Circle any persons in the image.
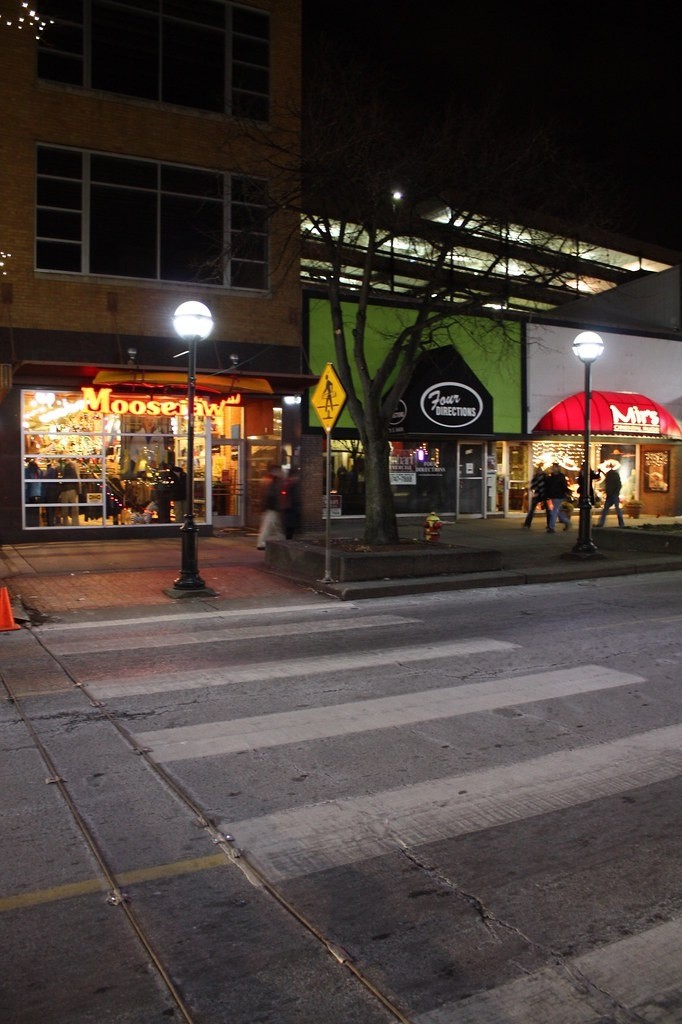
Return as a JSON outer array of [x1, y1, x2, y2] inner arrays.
[[592, 463, 625, 527], [578, 462, 600, 505], [546, 463, 571, 534], [256, 465, 303, 551], [25, 459, 186, 526], [524, 465, 551, 529]]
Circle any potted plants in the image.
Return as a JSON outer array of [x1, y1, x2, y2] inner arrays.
[[624, 500, 643, 520], [558, 502, 574, 523]]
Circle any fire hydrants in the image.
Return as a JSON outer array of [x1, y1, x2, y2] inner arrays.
[[421, 512, 443, 543]]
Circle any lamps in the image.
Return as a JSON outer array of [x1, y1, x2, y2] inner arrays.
[[230, 353, 239, 370], [127, 348, 138, 364]]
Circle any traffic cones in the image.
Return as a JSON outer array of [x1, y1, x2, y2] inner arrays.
[[1, 585, 22, 631]]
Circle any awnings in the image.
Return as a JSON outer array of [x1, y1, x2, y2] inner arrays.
[[533, 391, 682, 439], [11, 363, 321, 396]]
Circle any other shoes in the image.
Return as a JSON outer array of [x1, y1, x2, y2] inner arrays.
[[562, 523, 572, 532], [547, 528, 556, 533], [522, 524, 532, 529], [257, 544, 266, 550]]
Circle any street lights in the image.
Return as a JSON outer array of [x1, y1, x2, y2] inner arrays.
[[567, 330, 606, 554], [171, 300, 216, 598]]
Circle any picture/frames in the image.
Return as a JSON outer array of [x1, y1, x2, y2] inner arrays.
[[231, 424, 240, 460]]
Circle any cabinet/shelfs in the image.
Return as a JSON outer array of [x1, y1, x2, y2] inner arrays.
[[194, 478, 206, 504]]
